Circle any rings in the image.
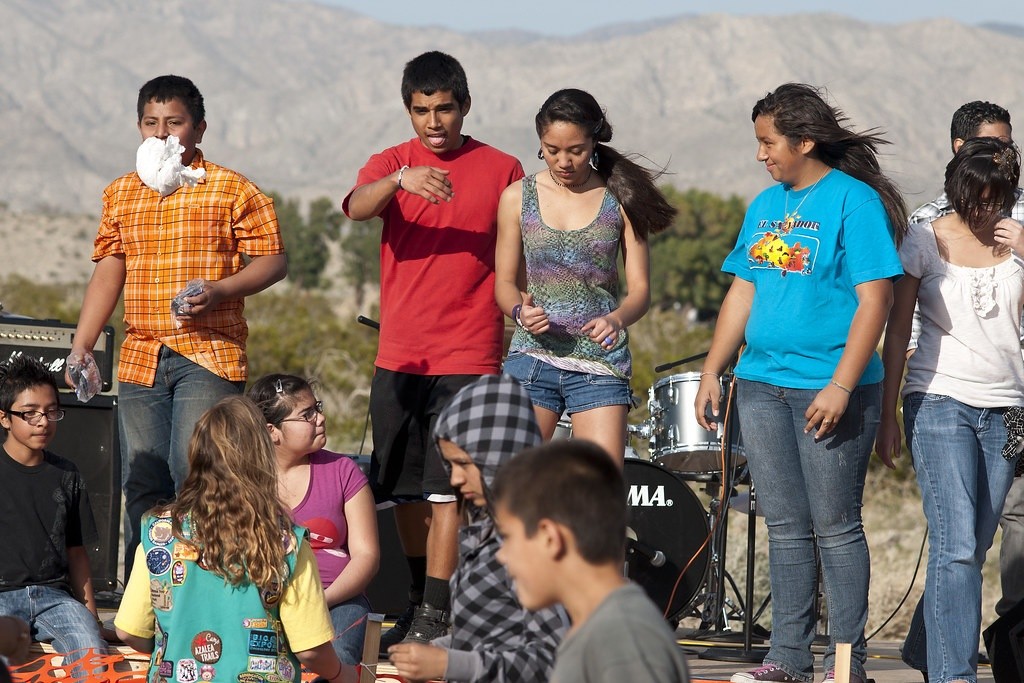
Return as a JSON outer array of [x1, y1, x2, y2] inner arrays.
[[823, 418, 831, 428], [604, 337, 613, 346]]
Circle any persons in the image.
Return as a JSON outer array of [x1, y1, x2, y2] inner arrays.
[[388, 374, 572, 683], [491, 437, 691, 683], [494, 86, 679, 470], [342, 50, 525, 660], [63, 75, 287, 584], [905, 101, 1024, 683], [0, 358, 107, 683], [113, 396, 359, 683], [249, 374, 379, 683], [694, 85, 907, 683], [875, 136, 1024, 683]]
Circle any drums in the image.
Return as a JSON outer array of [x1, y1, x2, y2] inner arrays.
[[621, 456, 712, 624], [648, 371, 747, 475], [549, 418, 632, 446]]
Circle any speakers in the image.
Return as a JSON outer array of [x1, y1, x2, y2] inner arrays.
[[47, 386, 122, 593]]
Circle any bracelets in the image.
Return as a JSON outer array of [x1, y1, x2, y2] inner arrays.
[[399, 165, 409, 190], [329, 662, 341, 680], [700, 372, 720, 379], [831, 381, 851, 395], [512, 304, 529, 332]]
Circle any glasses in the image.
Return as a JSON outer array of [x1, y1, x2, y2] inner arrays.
[[3, 410, 66, 423], [281, 401, 323, 422], [960, 197, 1004, 213]]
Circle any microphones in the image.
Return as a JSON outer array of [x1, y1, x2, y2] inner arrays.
[[627, 538, 666, 568]]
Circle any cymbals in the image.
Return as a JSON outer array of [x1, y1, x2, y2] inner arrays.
[[730, 492, 765, 517], [502, 325, 515, 358]]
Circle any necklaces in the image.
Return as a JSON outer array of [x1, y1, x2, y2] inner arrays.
[[550, 164, 592, 188], [782, 165, 831, 231]]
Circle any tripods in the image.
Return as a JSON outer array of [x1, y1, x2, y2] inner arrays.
[[668, 475, 772, 642]]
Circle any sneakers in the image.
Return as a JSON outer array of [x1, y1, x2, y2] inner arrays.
[[730, 665, 804, 683], [821, 669, 864, 683], [378, 605, 418, 658], [400, 603, 452, 645]]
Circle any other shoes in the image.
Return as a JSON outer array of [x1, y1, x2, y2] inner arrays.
[[975, 651, 991, 666]]
